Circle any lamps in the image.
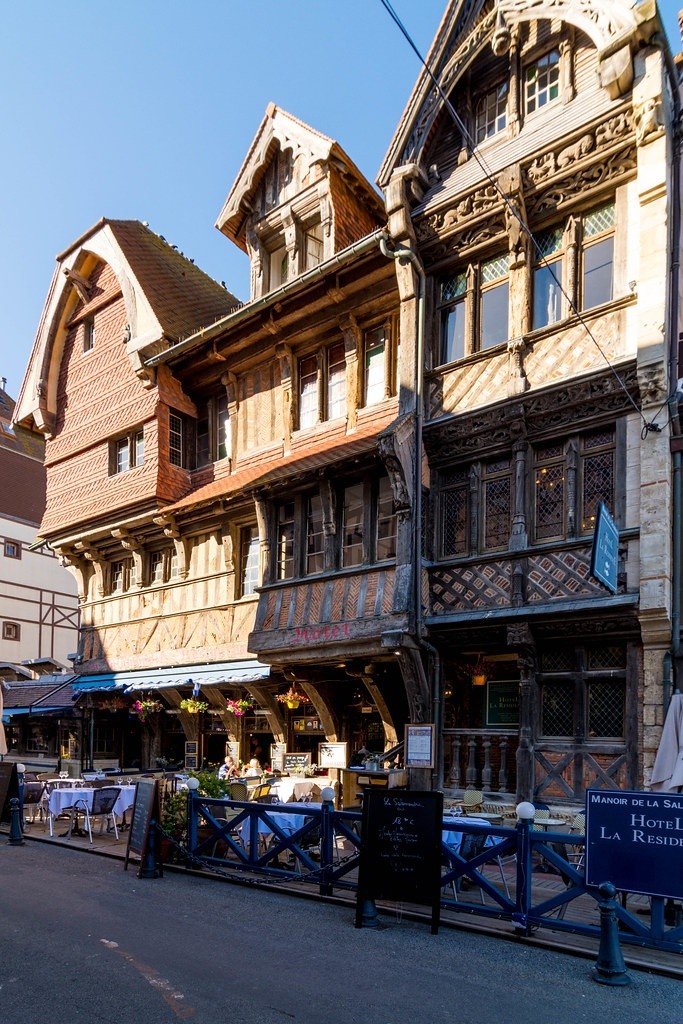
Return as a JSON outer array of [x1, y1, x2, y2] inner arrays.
[[187, 778, 200, 789], [516, 802, 536, 819], [17, 764, 26, 773], [321, 787, 335, 801]]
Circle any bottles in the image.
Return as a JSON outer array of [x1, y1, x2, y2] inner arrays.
[[96, 768, 102, 776]]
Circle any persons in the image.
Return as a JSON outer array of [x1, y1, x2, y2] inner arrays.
[[219, 756, 240, 782], [241, 759, 263, 778]]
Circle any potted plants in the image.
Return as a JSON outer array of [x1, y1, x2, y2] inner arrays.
[[98, 698, 127, 712], [159, 767, 232, 861], [180, 698, 208, 714]]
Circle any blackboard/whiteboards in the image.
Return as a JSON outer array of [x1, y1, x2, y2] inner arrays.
[[281, 752, 311, 773], [317, 742, 347, 769], [271, 760, 282, 772], [128, 777, 162, 858], [0, 761, 25, 831], [226, 742, 239, 769], [403, 722, 436, 768], [270, 743, 286, 759], [185, 756, 198, 768], [359, 785, 443, 906], [184, 741, 199, 754], [585, 790, 683, 902]]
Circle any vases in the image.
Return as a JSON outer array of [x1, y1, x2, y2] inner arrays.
[[287, 701, 300, 709], [147, 706, 155, 713]]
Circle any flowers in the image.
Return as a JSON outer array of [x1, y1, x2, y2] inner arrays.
[[275, 686, 310, 704], [240, 760, 250, 772], [133, 697, 164, 723], [225, 698, 255, 716]]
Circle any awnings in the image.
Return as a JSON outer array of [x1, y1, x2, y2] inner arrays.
[[2, 707, 66, 723]]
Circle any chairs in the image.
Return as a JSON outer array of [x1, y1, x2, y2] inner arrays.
[[229, 776, 585, 915], [23, 766, 186, 844]]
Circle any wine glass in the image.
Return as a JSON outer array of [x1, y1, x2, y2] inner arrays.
[[117, 777, 123, 787], [450, 805, 462, 822], [60, 770, 69, 779], [73, 780, 85, 789], [127, 777, 132, 787], [270, 797, 283, 806], [301, 792, 313, 805]]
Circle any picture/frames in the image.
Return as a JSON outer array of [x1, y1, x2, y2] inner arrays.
[[317, 743, 348, 770]]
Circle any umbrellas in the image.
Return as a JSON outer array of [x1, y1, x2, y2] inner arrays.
[[649, 689, 683, 792]]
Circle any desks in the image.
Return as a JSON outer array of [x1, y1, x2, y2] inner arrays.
[[47, 788, 105, 837], [83, 774, 104, 781], [240, 800, 339, 872], [46, 778, 85, 789], [103, 784, 137, 831], [247, 783, 263, 790], [443, 816, 491, 892], [535, 818, 565, 872]]
